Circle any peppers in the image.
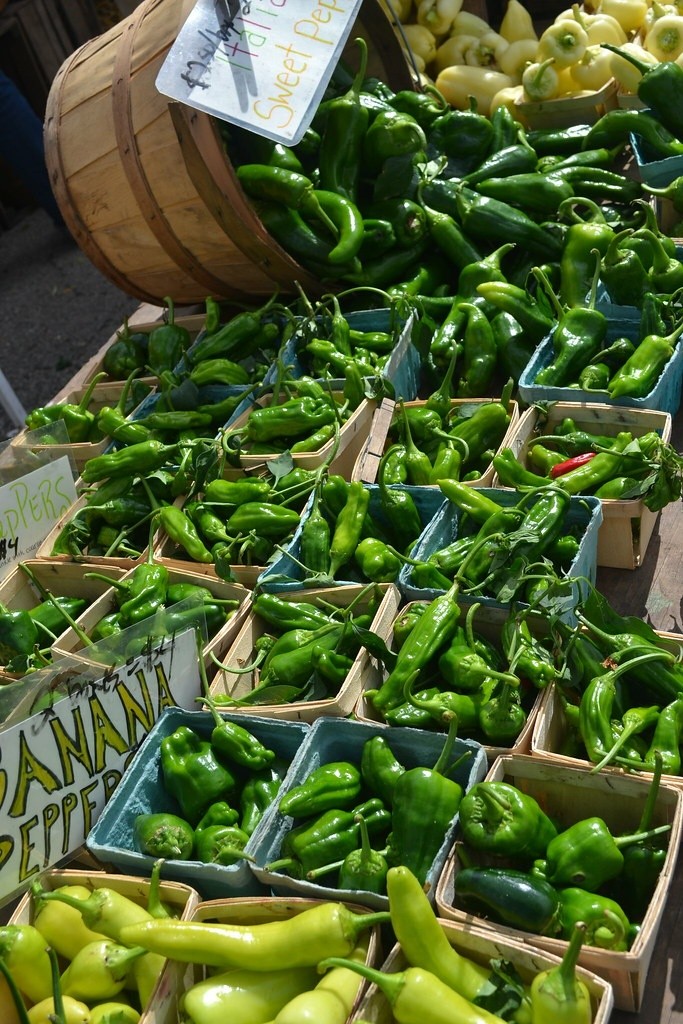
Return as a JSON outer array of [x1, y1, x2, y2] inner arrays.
[[0, 0, 683, 1024]]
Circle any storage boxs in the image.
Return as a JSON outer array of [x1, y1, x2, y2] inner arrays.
[[0, 41, 683, 1024]]
